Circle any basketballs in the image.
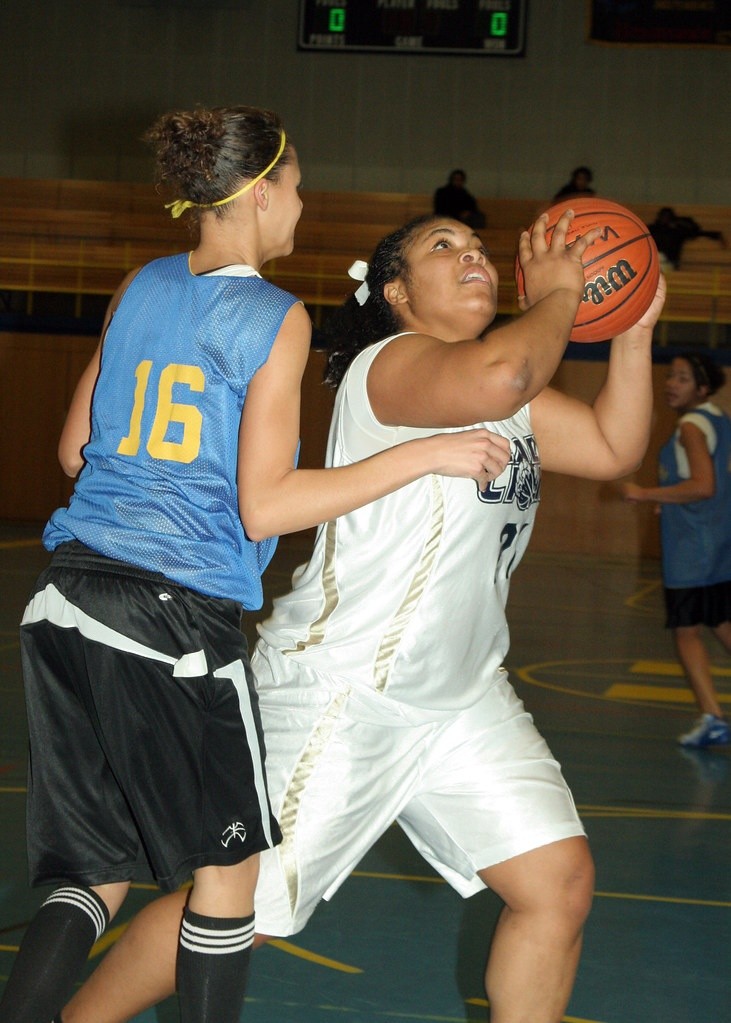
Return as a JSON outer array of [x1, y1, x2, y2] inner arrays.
[[512, 195, 660, 344]]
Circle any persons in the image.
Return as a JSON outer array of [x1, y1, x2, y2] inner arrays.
[[45, 216, 666, 1023], [432, 169, 485, 229], [618, 350, 731, 747], [0, 105, 510, 1023], [647, 207, 683, 270], [550, 166, 595, 204]]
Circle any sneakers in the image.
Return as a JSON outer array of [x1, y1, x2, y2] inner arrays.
[[677, 713, 731, 746]]
